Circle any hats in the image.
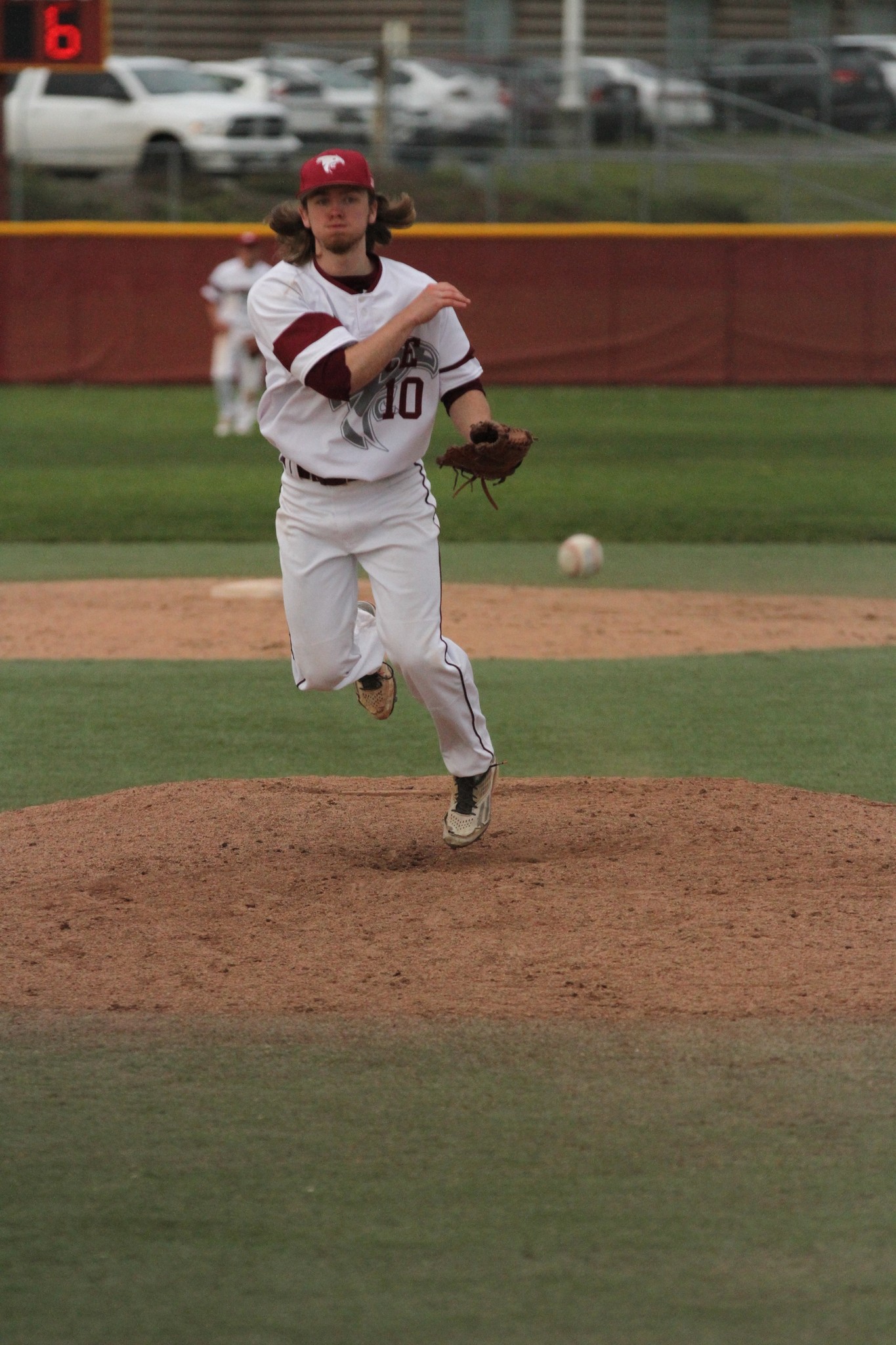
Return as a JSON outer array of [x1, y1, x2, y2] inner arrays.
[[240, 231, 259, 245], [300, 149, 376, 194]]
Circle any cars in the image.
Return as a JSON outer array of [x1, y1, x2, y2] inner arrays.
[[684, 36, 896, 137], [0, 54, 305, 180], [195, 54, 511, 148], [511, 56, 714, 147]]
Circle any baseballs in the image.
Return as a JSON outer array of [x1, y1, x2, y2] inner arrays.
[[558, 533, 605, 582]]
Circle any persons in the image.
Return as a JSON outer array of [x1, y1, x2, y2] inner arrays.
[[247, 150, 532, 846], [202, 229, 273, 438]]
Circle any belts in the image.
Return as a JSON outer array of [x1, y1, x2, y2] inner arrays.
[[282, 457, 358, 485]]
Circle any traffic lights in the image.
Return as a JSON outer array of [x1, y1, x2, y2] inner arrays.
[[0, 0, 114, 75]]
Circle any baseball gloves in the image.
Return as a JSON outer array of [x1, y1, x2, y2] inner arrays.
[[436, 419, 535, 482], [246, 335, 261, 360]]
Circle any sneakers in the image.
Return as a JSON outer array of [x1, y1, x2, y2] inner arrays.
[[442, 758, 507, 848], [353, 601, 397, 720]]
